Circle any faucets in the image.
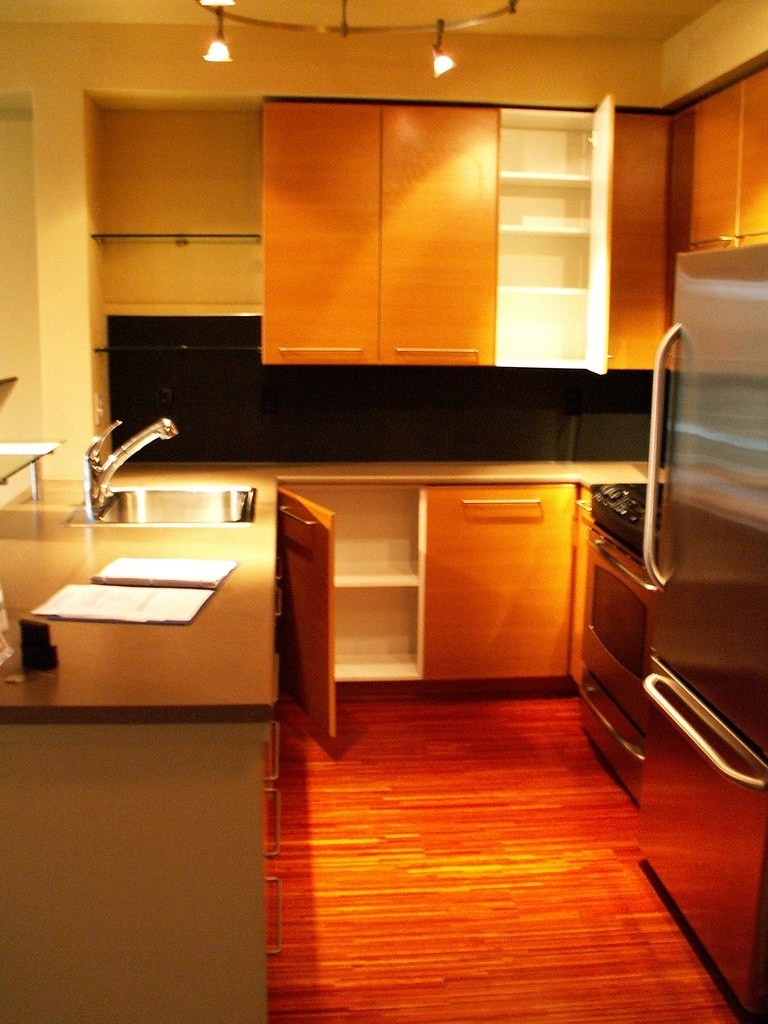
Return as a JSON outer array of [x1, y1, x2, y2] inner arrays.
[[82, 417, 180, 510]]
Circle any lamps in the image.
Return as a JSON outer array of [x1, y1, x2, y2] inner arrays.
[[429, 20, 456, 79], [203, 6, 234, 62]]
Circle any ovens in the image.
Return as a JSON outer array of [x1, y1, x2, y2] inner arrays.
[[578, 481, 663, 804]]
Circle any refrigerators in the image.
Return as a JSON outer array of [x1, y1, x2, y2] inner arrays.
[[636, 244, 767, 1024]]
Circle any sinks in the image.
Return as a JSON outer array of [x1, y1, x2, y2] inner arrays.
[[59, 483, 258, 530]]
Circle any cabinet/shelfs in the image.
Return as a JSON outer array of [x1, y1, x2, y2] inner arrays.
[[668, 67, 768, 245], [495, 92, 669, 375], [260, 103, 502, 366], [278, 483, 582, 738]]
